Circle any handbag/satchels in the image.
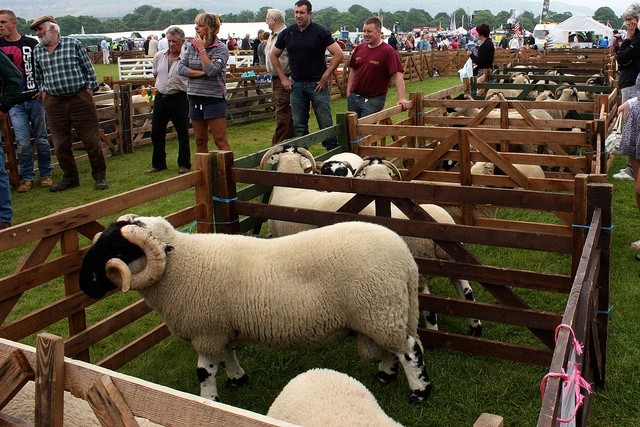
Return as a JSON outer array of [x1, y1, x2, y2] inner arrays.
[[605, 129, 622, 156]]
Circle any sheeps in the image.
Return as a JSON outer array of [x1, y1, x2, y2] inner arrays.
[[94, 80, 161, 120], [489, 50, 609, 147], [319, 150, 365, 180], [446, 92, 565, 171], [442, 141, 497, 174], [77, 213, 433, 405], [468, 155, 548, 198], [352, 155, 482, 336], [258, 143, 374, 238]]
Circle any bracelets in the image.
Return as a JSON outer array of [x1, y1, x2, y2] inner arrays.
[[198, 49, 206, 55]]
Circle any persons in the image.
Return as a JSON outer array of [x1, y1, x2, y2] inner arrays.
[[237, 36, 242, 49], [269, 0, 344, 152], [177, 13, 230, 153], [347, 17, 411, 138], [143, 36, 153, 55], [388, 33, 482, 51], [252, 29, 265, 66], [529, 34, 535, 48], [1, 10, 53, 193], [509, 35, 524, 49], [257, 32, 270, 65], [600, 36, 609, 48], [30, 17, 109, 192], [467, 24, 496, 77], [543, 29, 554, 49], [612, 3, 640, 181], [242, 33, 252, 50], [616, 74, 640, 258], [610, 31, 626, 73], [266, 9, 294, 146], [0, 49, 22, 230], [148, 35, 159, 55], [112, 37, 134, 51], [498, 34, 508, 48], [158, 32, 168, 51], [101, 36, 111, 65], [593, 34, 601, 47], [227, 35, 237, 50], [145, 26, 191, 174]]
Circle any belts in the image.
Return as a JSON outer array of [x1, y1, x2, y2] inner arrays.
[[272, 74, 290, 79]]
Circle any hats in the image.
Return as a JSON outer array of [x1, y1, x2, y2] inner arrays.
[[30, 16, 56, 30]]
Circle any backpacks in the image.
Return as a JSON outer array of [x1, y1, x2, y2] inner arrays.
[[615, 35, 623, 48]]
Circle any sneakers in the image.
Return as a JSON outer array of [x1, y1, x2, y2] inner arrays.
[[50, 182, 67, 192], [631, 239, 640, 249], [17, 181, 34, 193], [146, 168, 157, 173], [40, 177, 53, 187], [0, 223, 11, 230], [178, 167, 188, 173], [613, 171, 635, 181], [97, 179, 107, 188]]
